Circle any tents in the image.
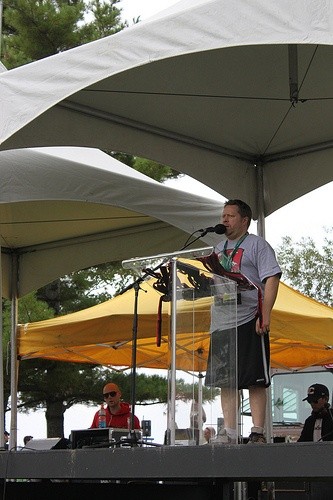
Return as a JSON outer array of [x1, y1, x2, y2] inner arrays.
[[0, 0, 333, 451]]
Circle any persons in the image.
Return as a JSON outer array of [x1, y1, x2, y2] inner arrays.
[[4, 430, 10, 450], [89, 383, 141, 430], [204, 199, 283, 443], [23, 436, 33, 446], [203, 427, 216, 444], [296, 383, 333, 443]]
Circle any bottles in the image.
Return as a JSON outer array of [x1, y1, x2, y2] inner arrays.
[[98, 408, 106, 428]]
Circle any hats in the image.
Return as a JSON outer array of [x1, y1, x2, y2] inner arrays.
[[302, 383, 329, 401]]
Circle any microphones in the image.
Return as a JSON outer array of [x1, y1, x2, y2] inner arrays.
[[199, 224, 227, 234]]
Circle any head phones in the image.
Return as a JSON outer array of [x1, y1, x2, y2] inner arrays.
[[324, 403, 330, 409]]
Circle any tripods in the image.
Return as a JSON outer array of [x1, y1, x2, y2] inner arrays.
[[81, 232, 206, 450]]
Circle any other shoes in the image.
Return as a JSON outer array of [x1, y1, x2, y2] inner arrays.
[[212, 429, 239, 444], [246, 432, 266, 444]]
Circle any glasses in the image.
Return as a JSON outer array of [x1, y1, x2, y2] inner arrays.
[[307, 396, 325, 403], [103, 391, 117, 397]]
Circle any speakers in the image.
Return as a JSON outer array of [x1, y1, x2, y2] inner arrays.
[[20, 437, 71, 449]]
